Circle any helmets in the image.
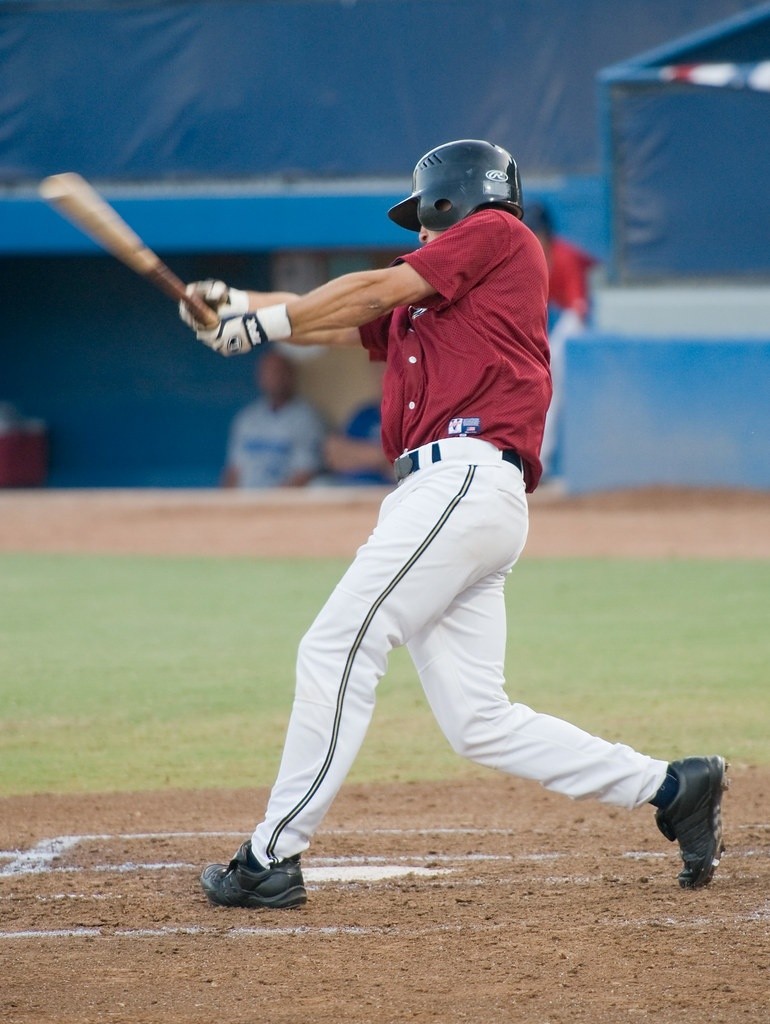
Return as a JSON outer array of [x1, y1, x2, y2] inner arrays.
[[386, 137, 523, 234]]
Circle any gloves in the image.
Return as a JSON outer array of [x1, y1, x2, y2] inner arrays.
[[178, 278, 247, 331], [195, 303, 293, 358]]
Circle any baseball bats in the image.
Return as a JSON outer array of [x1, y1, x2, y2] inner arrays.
[[35, 172, 217, 331]]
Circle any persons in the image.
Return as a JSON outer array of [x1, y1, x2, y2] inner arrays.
[[179, 140, 727, 908], [222, 354, 329, 488], [305, 406, 400, 487]]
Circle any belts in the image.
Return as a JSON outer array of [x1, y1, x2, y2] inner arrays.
[[393, 442, 523, 482]]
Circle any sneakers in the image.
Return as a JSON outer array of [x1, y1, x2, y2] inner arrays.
[[654, 755, 730, 889], [198, 840, 309, 912]]
